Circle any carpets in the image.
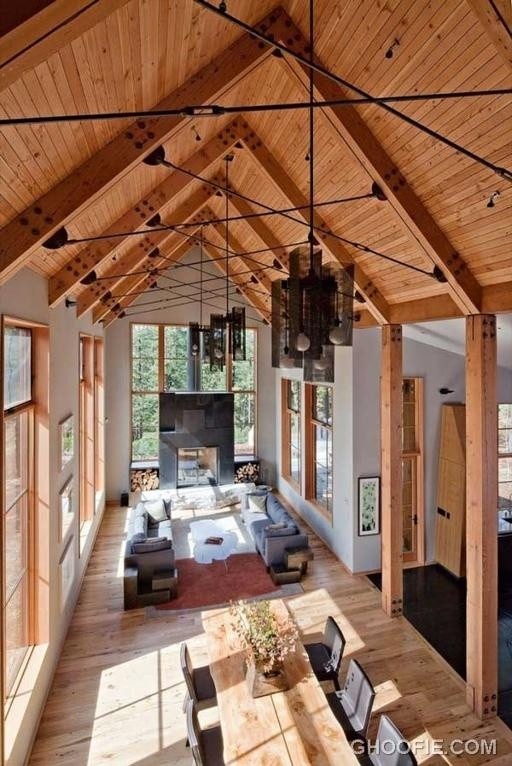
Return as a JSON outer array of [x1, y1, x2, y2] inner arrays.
[[144, 558, 306, 620]]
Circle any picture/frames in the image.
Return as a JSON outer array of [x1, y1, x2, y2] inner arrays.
[[58, 412, 76, 472], [58, 535, 76, 613], [58, 475, 77, 544], [358, 476, 381, 536]]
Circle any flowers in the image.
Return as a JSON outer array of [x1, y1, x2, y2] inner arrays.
[[233, 462, 260, 484], [216, 595, 301, 661]]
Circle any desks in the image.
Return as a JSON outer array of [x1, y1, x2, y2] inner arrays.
[[198, 597, 362, 766]]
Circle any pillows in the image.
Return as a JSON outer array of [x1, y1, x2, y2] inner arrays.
[[247, 493, 268, 514], [265, 522, 287, 529], [143, 500, 170, 525]]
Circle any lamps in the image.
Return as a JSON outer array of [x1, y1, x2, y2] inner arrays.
[[209, 157, 247, 371], [270, 0, 357, 386]]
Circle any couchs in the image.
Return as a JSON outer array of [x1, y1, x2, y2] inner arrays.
[[123, 500, 175, 611], [239, 490, 308, 574]]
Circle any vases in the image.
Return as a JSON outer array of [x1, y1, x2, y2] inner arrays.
[[242, 657, 292, 699]]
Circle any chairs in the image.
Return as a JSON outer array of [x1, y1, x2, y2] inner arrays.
[[324, 657, 376, 743], [182, 699, 224, 766], [303, 616, 347, 690], [179, 641, 216, 713], [357, 714, 419, 766]]
